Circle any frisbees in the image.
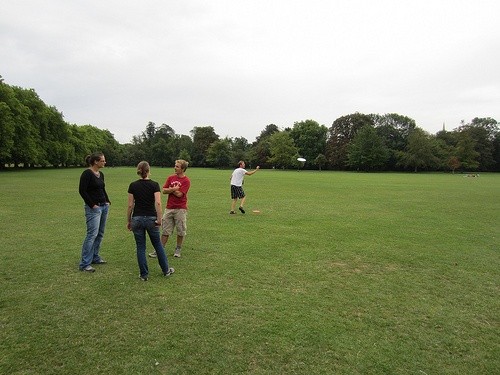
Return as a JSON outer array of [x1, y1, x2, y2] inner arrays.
[[297, 158, 307, 162]]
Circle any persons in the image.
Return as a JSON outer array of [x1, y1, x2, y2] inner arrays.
[[148, 160, 190, 257], [79, 152, 112, 272], [126, 162, 175, 281], [230, 161, 259, 214]]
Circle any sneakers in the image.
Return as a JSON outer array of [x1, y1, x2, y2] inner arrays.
[[148, 249, 157, 257], [174, 247, 182, 257], [82, 265, 95, 272], [230, 210, 235, 214], [138, 274, 148, 281], [164, 267, 175, 276], [239, 206, 245, 213], [97, 260, 107, 264]]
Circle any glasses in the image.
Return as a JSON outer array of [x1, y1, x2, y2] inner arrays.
[[99, 158, 106, 163]]
